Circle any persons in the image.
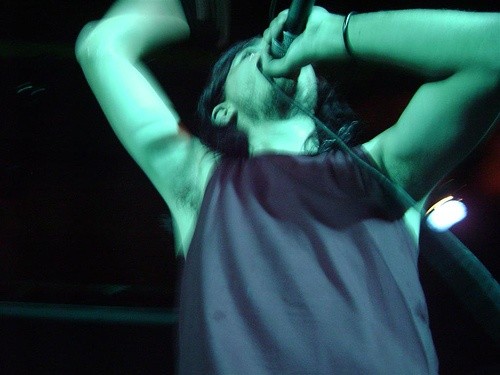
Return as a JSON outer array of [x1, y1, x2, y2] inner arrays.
[[75, 0, 500, 375]]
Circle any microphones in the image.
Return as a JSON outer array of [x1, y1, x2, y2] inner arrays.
[[270, 0, 315, 58]]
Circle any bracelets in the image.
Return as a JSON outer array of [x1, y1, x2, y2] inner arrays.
[[343, 12, 368, 63]]
